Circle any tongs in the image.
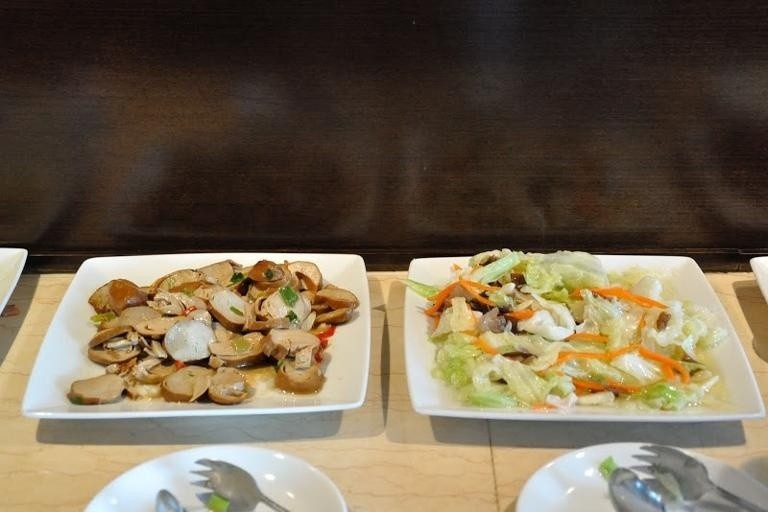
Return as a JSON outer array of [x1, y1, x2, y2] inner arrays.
[[151, 454, 286, 512], [603, 443, 763, 511]]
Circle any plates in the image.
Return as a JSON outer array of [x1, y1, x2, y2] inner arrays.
[[509, 440, 768, 512], [748, 253, 766, 305], [0, 243, 27, 313], [79, 440, 347, 511], [20, 253, 373, 422], [402, 251, 763, 426]]
